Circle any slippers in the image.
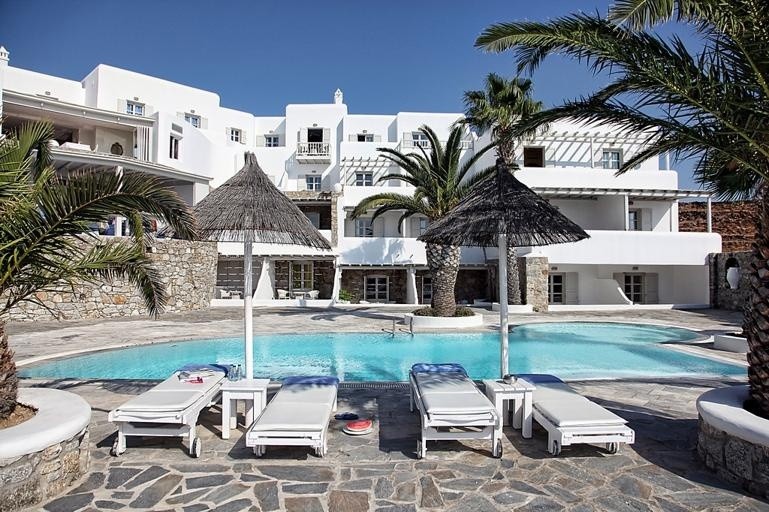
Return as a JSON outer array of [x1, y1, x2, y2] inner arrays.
[[334, 412, 359, 420]]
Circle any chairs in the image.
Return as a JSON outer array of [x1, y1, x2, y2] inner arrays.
[[277, 289, 289, 300], [220, 289, 230, 299], [307, 290, 319, 299]]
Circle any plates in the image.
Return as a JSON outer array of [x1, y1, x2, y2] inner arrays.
[[342, 425, 374, 435]]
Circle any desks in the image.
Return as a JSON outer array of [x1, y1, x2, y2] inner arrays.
[[230, 290, 242, 300], [293, 292, 305, 300]]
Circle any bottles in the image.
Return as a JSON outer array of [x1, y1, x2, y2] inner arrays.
[[228, 363, 242, 381]]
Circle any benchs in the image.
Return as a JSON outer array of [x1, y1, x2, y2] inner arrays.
[[410, 363, 636, 460], [107, 362, 340, 457]]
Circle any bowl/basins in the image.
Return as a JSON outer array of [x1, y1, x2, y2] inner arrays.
[[503, 373, 517, 384]]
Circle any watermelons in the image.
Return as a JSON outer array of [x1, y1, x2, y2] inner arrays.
[[346, 420, 373, 431]]
